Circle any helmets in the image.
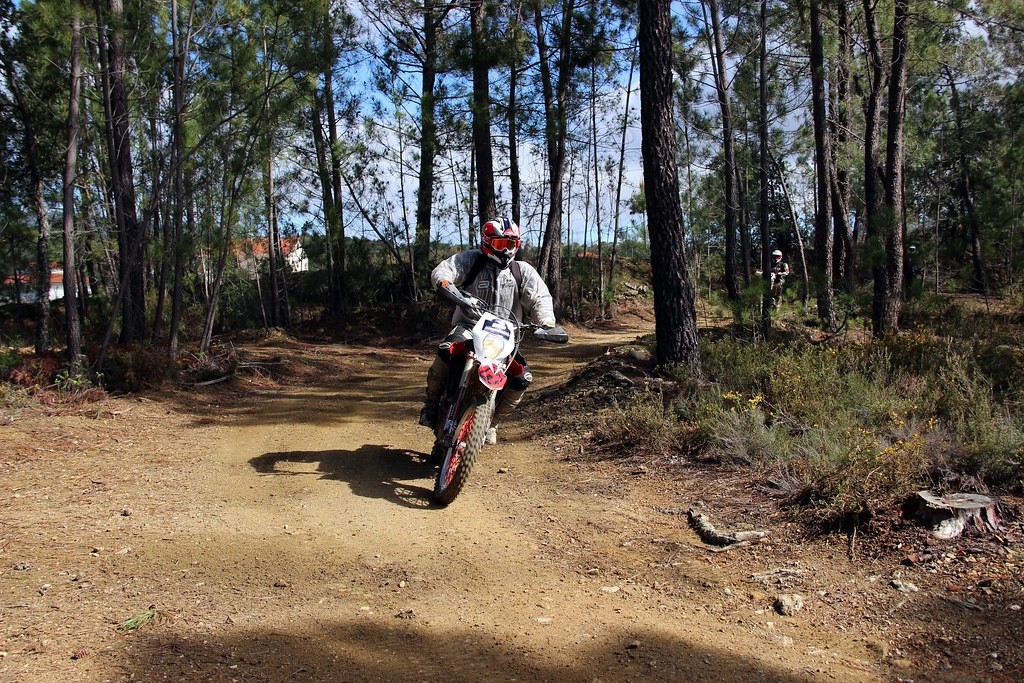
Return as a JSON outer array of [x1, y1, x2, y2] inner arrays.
[[481, 216, 520, 270], [772, 250, 782, 263]]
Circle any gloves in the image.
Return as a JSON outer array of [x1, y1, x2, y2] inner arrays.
[[541, 325, 554, 330], [438, 281, 452, 287]]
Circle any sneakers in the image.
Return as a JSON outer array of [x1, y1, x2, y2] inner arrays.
[[485, 422, 498, 445], [419, 398, 441, 427]]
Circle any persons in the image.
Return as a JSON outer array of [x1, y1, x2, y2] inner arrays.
[[756, 250, 789, 307], [417, 217, 556, 446], [902, 245, 918, 275]]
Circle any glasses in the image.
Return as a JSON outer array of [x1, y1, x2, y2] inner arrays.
[[491, 239, 520, 253]]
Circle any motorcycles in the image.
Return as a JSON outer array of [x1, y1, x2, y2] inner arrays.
[[420, 285, 570, 506], [755, 270, 782, 313]]
